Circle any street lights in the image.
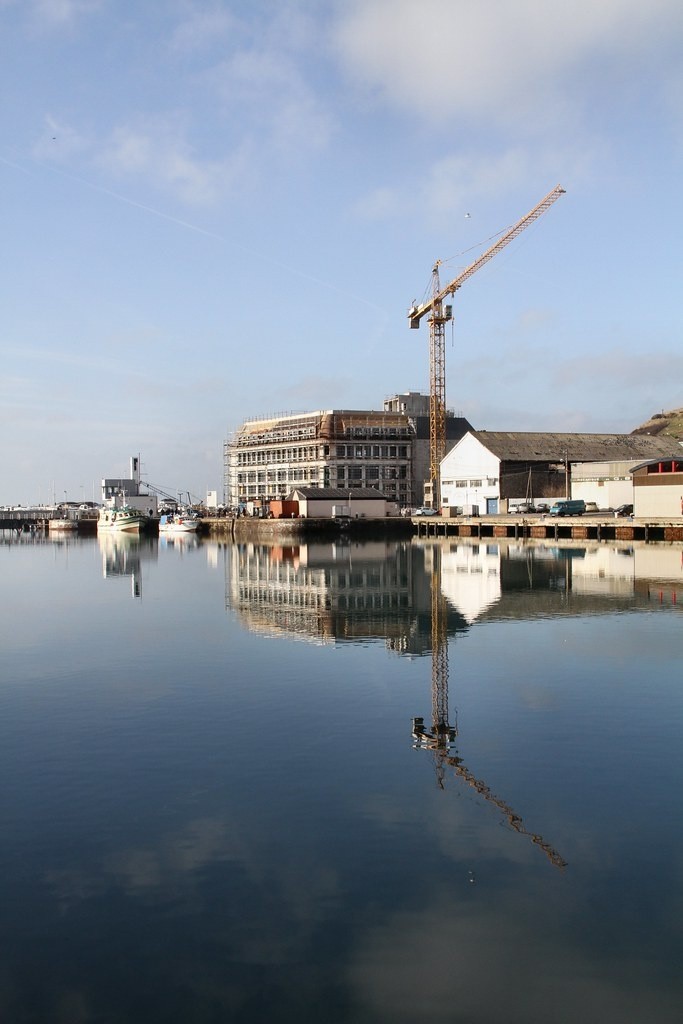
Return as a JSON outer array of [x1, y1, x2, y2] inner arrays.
[[64, 491, 67, 516], [178, 493, 183, 503], [475, 490, 478, 516], [560, 459, 569, 500], [349, 493, 351, 508]]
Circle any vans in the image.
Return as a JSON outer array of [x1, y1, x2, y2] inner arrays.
[[548, 499, 586, 517]]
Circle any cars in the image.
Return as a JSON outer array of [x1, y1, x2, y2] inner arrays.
[[416, 507, 437, 516], [584, 502, 598, 512], [519, 503, 533, 513], [508, 504, 518, 513], [536, 503, 550, 512], [187, 509, 204, 518], [614, 504, 635, 516]]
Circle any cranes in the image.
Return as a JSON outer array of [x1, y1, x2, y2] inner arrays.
[[412, 542, 569, 874], [407, 182, 566, 506]]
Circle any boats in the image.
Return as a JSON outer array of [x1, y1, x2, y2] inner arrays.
[[97, 488, 151, 537], [157, 514, 203, 532]]
